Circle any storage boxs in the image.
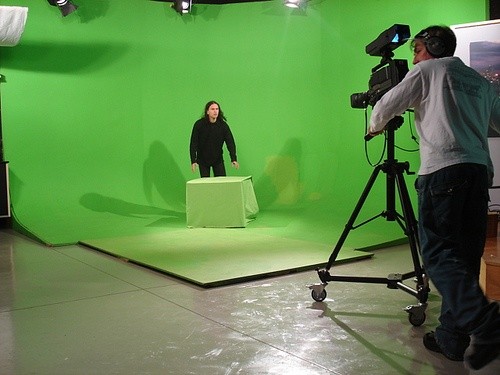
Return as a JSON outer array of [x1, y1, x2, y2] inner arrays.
[[186, 176, 259, 228]]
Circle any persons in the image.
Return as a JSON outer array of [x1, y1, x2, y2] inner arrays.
[[190, 101, 239, 179], [368, 24, 500, 371]]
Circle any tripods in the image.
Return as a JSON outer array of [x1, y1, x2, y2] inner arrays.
[[306, 116, 431, 327]]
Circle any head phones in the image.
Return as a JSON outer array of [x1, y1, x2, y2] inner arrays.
[[416, 30, 448, 57]]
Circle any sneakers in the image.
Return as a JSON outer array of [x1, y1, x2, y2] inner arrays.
[[463, 322, 500, 369], [424, 326, 468, 360]]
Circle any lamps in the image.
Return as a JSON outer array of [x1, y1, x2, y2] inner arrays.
[[48, 0, 301, 17]]
